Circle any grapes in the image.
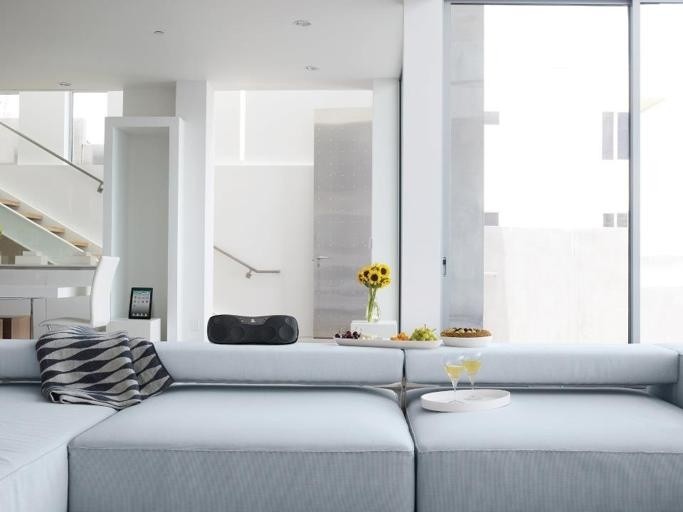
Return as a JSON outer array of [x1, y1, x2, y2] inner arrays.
[[410, 324, 436, 341]]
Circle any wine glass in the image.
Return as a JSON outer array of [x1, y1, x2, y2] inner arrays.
[[444, 348, 483, 405]]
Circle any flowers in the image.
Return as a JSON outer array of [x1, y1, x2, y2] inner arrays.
[[358, 262, 391, 323]]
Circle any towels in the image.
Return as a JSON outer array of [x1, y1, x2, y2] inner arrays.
[[36, 326, 176, 411]]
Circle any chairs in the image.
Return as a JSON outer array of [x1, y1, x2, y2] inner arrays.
[[38, 255, 119, 331]]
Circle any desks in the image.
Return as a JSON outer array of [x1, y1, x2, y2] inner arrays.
[[0, 285, 91, 338]]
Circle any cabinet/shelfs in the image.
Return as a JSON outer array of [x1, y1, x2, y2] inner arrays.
[[109, 319, 163, 341]]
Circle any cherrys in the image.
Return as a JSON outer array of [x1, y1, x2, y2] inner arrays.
[[335, 330, 360, 338]]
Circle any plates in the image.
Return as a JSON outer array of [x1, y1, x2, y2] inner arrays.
[[383, 339, 443, 348], [333, 336, 383, 346], [440, 334, 493, 348]]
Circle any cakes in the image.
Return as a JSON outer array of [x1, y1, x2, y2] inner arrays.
[[441, 328, 491, 337]]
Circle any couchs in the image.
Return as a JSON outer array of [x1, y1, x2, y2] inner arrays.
[[0, 337, 682, 510]]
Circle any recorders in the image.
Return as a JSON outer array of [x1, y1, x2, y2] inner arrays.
[[207, 314, 298, 345]]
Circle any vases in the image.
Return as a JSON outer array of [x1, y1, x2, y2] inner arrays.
[[351, 320, 397, 340]]
